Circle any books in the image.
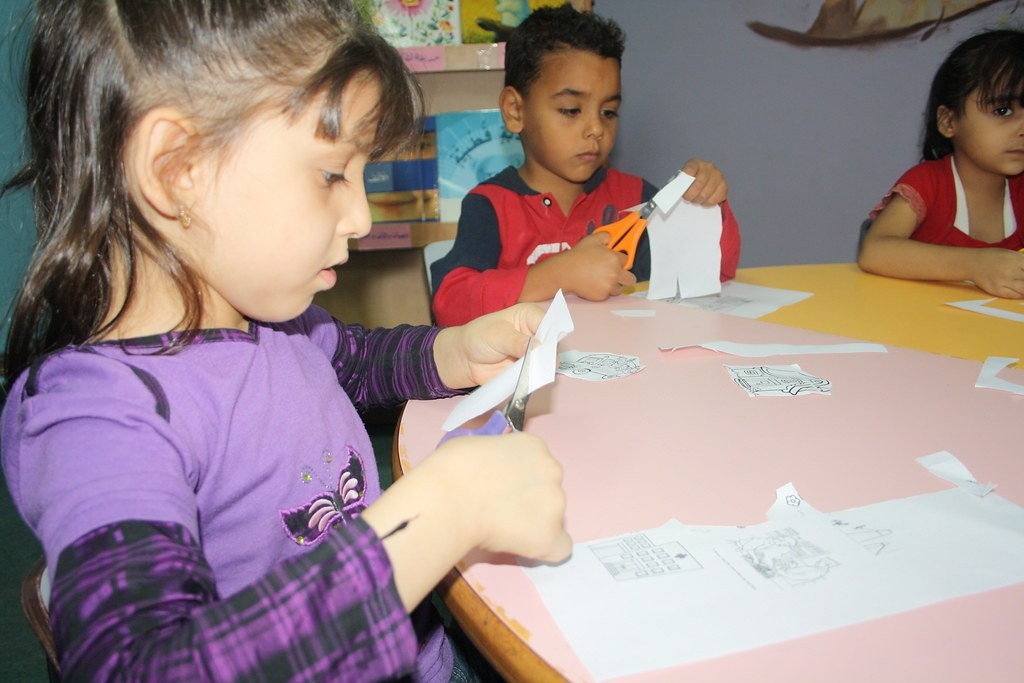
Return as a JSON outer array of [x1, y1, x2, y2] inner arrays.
[[357, 0, 597, 48], [365, 106, 525, 224]]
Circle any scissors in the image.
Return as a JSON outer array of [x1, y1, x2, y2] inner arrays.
[[591, 168, 682, 270], [435, 334, 536, 445]]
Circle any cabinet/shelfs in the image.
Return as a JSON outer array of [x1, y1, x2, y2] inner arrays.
[[348, 41, 506, 253]]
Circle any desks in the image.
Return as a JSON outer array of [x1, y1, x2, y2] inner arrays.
[[392, 263, 1024, 683]]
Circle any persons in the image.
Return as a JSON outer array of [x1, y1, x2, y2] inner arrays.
[[0, 0, 573, 683], [857, 29, 1024, 299], [429, 0, 741, 328]]
